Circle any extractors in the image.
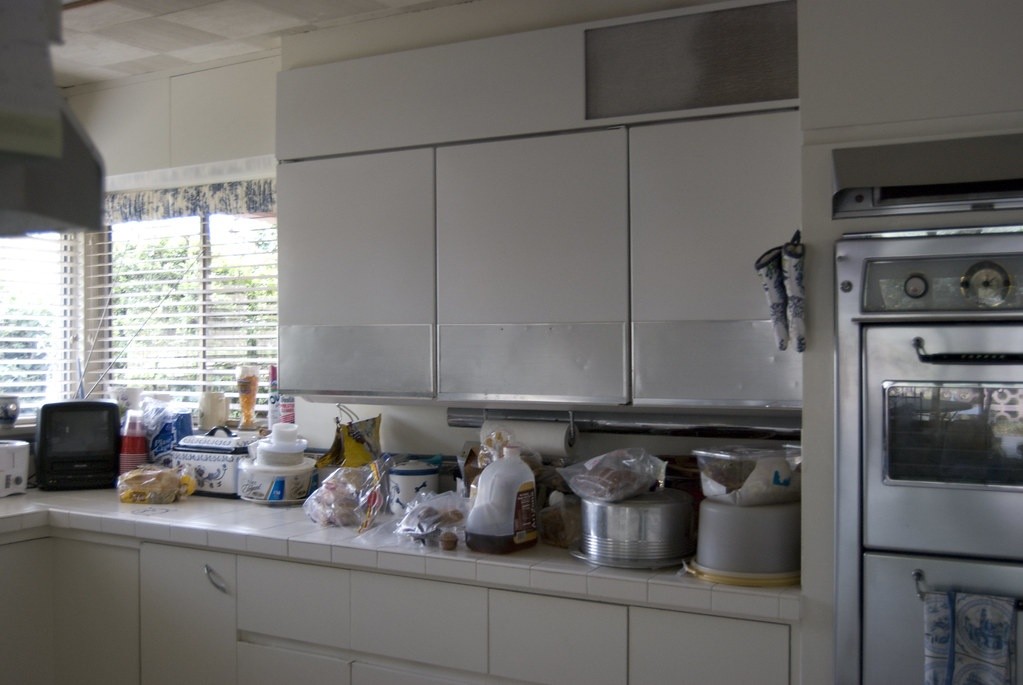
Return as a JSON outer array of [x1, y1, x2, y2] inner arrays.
[[2, 97, 109, 241]]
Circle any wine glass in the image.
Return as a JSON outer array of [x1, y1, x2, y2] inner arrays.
[[235, 364, 260, 430]]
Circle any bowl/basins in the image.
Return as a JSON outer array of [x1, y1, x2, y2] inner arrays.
[[567, 443, 801, 588]]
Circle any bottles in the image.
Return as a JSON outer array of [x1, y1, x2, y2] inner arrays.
[[268, 365, 295, 433]]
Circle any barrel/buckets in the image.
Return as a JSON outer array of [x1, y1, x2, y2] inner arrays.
[[465, 445, 537, 552]]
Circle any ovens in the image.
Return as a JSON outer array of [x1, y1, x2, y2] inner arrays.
[[829, 188, 1022, 563]]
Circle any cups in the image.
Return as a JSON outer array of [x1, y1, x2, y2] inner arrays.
[[389, 459, 439, 514], [0, 393, 21, 429], [106, 381, 192, 477], [198, 391, 230, 430]]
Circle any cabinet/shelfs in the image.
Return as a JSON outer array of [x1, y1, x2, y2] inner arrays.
[[0, 524, 799, 685], [628, 106, 803, 412], [275, 146, 436, 398], [432, 125, 631, 410]]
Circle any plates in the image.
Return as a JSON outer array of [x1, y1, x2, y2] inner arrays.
[[240, 496, 307, 505]]
